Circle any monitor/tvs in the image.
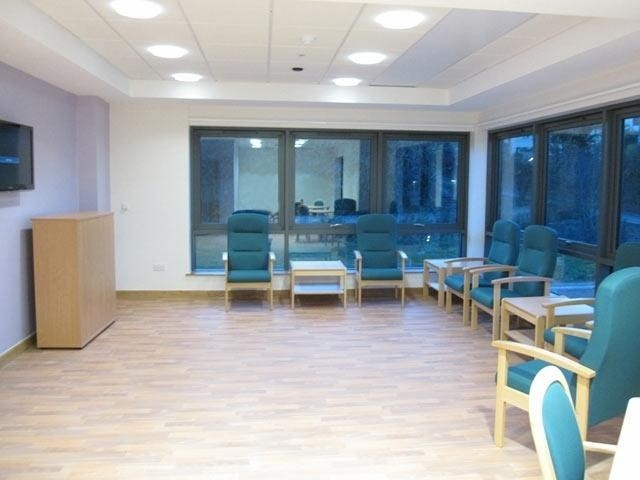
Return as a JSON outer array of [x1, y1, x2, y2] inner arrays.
[[0, 119, 35, 192]]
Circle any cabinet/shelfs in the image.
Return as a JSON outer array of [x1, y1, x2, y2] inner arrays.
[[28, 208, 118, 353]]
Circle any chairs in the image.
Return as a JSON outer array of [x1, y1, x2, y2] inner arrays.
[[488, 264, 640, 446], [332, 198, 357, 217], [351, 212, 408, 307], [442, 218, 522, 326], [220, 211, 276, 312], [233, 209, 274, 250], [311, 199, 325, 215], [469, 224, 563, 343], [541, 241, 640, 358], [526, 364, 617, 480]]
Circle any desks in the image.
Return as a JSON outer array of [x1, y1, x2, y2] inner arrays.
[[286, 241, 329, 260], [420, 256, 480, 309], [289, 259, 350, 313], [497, 294, 594, 356], [309, 210, 335, 216], [307, 204, 328, 210], [604, 396, 640, 480]]
